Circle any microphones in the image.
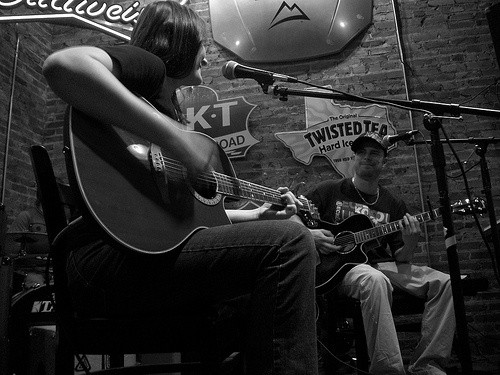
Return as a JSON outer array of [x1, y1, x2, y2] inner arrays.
[[383, 129, 419, 147], [221, 60, 298, 83]]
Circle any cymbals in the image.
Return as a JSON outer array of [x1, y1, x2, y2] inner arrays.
[[5, 232, 48, 244]]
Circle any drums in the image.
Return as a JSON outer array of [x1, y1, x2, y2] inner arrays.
[[5, 283, 57, 375]]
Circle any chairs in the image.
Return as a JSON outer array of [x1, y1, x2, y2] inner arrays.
[[29, 144, 261, 375], [317, 288, 424, 375]]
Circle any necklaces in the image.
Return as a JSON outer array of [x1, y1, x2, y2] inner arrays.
[[351, 180, 380, 205]]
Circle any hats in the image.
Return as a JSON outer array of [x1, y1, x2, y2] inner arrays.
[[351, 130, 389, 152]]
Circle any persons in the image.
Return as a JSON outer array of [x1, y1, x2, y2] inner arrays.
[[301, 131, 456, 375], [41, 1, 318, 375]]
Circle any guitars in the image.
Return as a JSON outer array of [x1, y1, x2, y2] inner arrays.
[[63, 91, 321, 256], [313, 196, 489, 288]]
